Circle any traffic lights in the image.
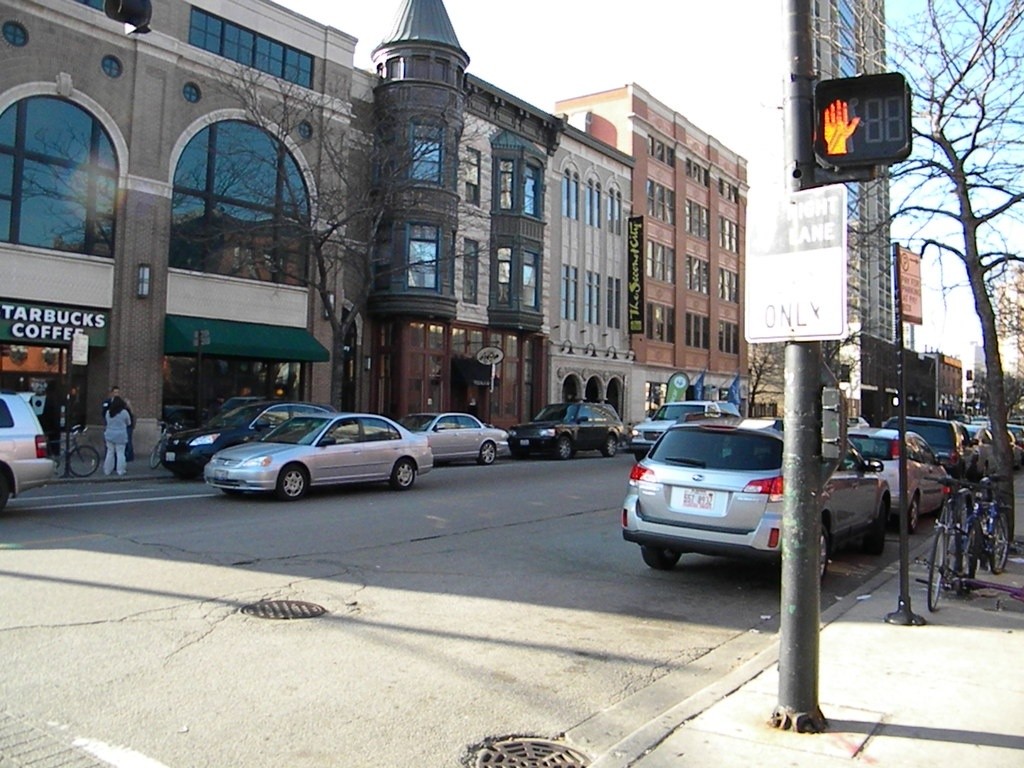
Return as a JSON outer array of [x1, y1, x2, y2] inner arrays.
[[810, 71, 914, 166]]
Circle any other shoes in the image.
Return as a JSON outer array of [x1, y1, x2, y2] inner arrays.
[[117, 470, 126, 476], [106, 469, 112, 475]]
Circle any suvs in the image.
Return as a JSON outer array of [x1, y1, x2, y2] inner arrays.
[[0, 390, 58, 516]]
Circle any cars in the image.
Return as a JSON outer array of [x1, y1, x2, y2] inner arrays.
[[506, 400, 629, 458], [621, 412, 889, 585], [620, 400, 1024, 485], [162, 402, 433, 501], [393, 410, 512, 469], [842, 428, 955, 534]]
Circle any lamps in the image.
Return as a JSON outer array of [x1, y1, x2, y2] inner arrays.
[[324, 290, 335, 319], [605, 345, 618, 359], [138, 262, 151, 299], [561, 339, 575, 355], [585, 343, 598, 357], [625, 348, 638, 362]]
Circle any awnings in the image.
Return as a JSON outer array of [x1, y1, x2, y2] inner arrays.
[[163, 315, 330, 362]]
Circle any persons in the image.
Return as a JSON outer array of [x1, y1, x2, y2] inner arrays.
[[848, 413, 871, 427], [101, 385, 136, 475]]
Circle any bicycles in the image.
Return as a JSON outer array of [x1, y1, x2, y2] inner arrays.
[[916, 474, 1017, 613], [43, 423, 101, 480], [148, 421, 184, 469]]
